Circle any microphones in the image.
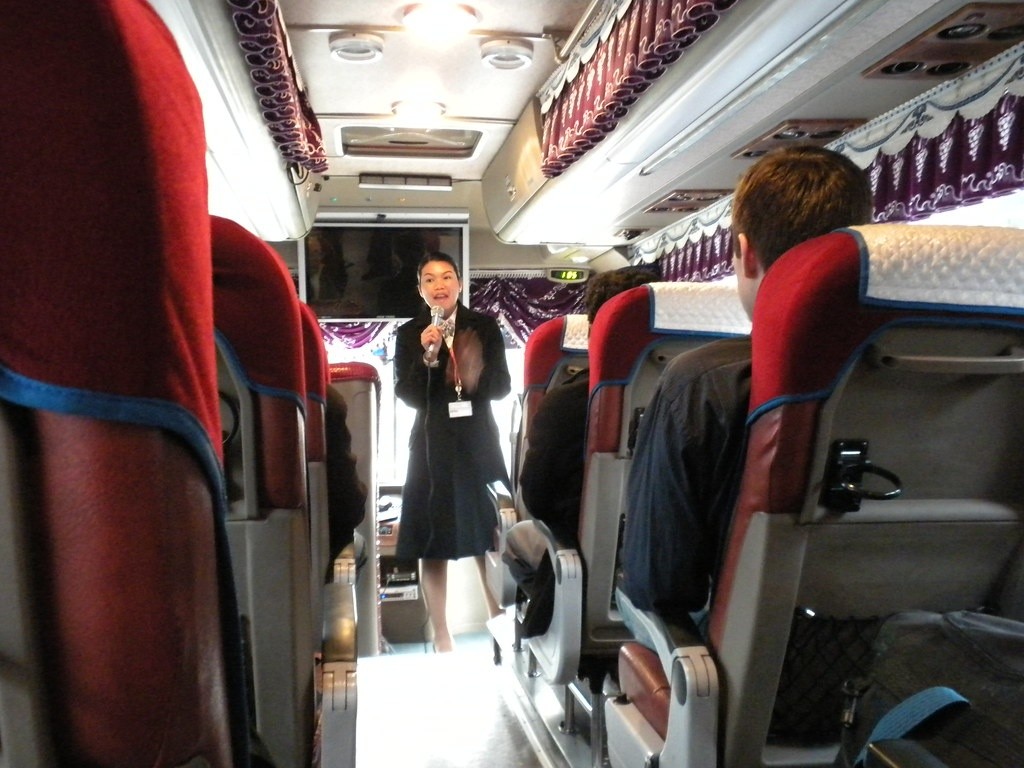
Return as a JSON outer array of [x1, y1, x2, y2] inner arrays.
[[429, 305, 445, 359]]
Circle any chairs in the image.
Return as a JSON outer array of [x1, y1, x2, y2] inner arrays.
[[296, 298, 331, 651], [603, 224, 1024, 768], [517, 282, 758, 738], [209, 215, 357, 767], [0, 0, 279, 768], [478, 312, 592, 678]]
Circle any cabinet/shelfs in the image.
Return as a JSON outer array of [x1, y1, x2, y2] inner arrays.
[[481, 96, 552, 234]]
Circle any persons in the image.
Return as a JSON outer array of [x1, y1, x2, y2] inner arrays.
[[392, 252, 511, 665], [623, 145, 875, 643], [501, 266, 664, 630], [326, 385, 368, 581]]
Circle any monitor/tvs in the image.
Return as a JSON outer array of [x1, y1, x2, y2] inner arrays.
[[297, 222, 472, 323]]
